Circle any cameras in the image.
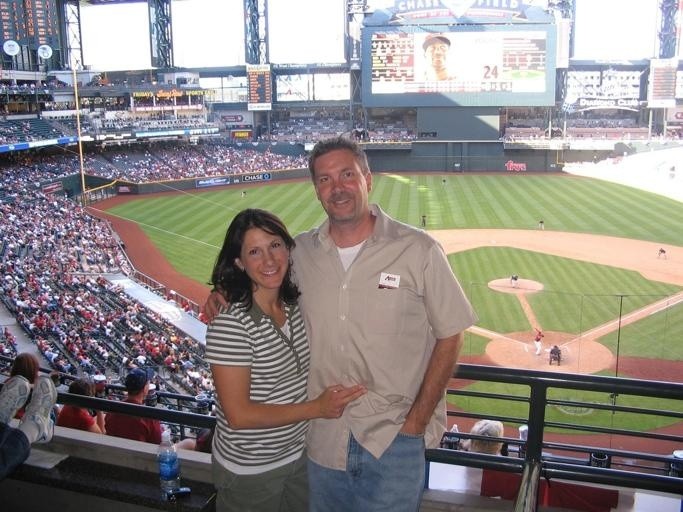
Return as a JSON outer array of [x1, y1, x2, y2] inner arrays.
[[51, 373, 78, 387]]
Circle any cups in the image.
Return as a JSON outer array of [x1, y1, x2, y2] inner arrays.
[[146, 382, 157, 398], [667, 450, 683, 477], [193, 393, 209, 408], [92, 374, 106, 393], [590, 452, 608, 468]]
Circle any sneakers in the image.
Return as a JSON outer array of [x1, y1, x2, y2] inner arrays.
[[0, 375, 30, 424], [20, 371, 57, 444]]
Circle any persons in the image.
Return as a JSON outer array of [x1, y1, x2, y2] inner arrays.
[[202, 207, 367, 511], [0, 373, 58, 480], [442, 180, 446, 186], [502, 112, 646, 139], [2, 178, 216, 457], [461, 419, 505, 457], [533, 331, 544, 356], [202, 134, 480, 511], [511, 274, 519, 287], [657, 247, 666, 260], [537, 218, 544, 230], [422, 214, 427, 227], [2, 75, 418, 183], [548, 344, 561, 366], [421, 34, 461, 83]]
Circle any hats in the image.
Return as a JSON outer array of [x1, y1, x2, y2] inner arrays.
[[125, 368, 154, 390]]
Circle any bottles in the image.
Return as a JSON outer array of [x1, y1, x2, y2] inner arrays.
[[156, 428, 182, 494], [518, 425, 529, 458], [448, 423, 460, 450]]
